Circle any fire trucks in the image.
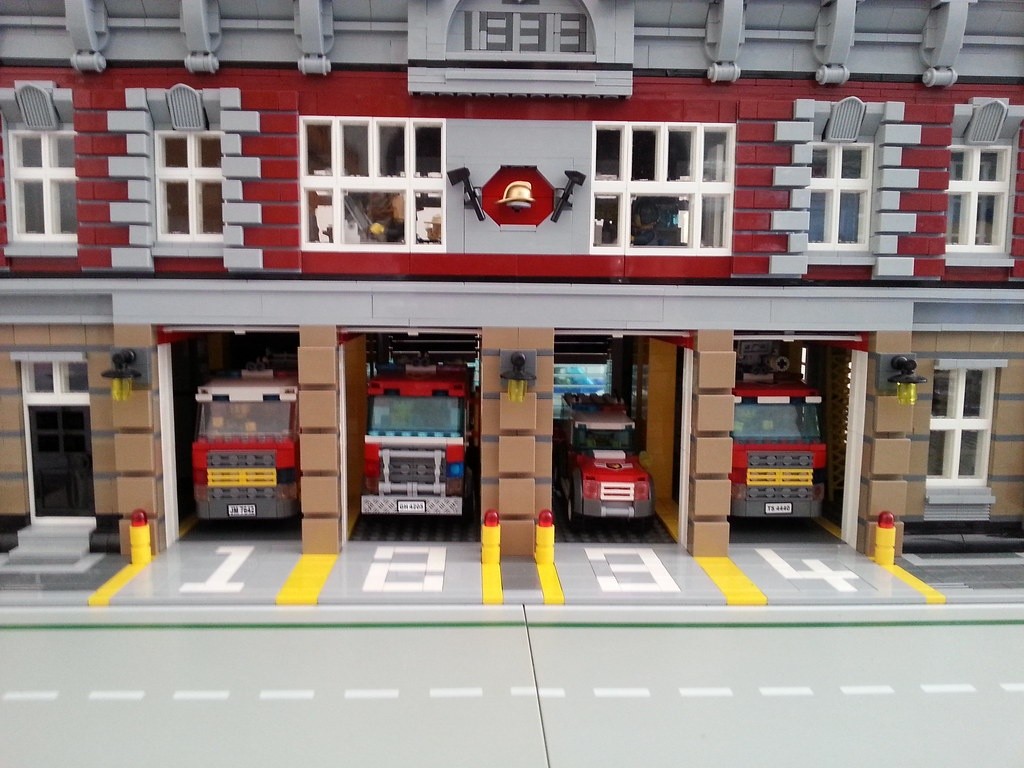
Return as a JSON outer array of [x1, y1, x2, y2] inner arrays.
[[192, 356, 300, 518], [731, 370, 829, 525], [361, 345, 480, 527], [559, 390, 653, 531]]
